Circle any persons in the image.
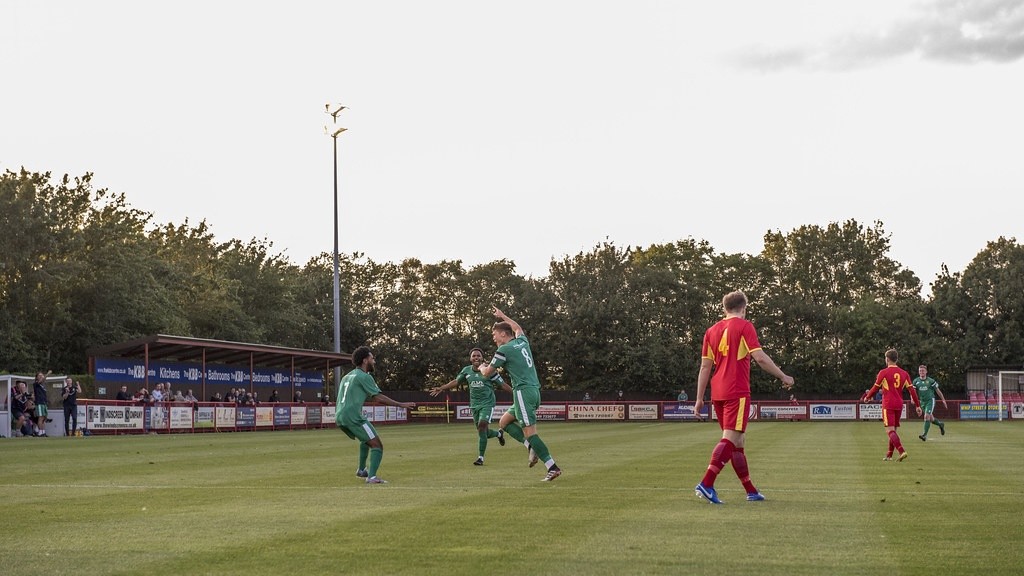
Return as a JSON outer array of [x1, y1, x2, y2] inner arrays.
[[615, 390, 626, 422], [294, 389, 305, 431], [269, 390, 280, 431], [336, 345, 416, 484], [225, 387, 262, 433], [478, 305, 562, 481], [582, 393, 592, 401], [429, 347, 513, 466], [859, 388, 883, 422], [789, 394, 801, 422], [678, 390, 689, 402], [62, 378, 82, 437], [864, 348, 922, 461], [909, 363, 948, 442], [316, 394, 331, 429], [116, 381, 198, 435], [4, 369, 53, 437], [694, 289, 794, 504], [210, 391, 222, 434]]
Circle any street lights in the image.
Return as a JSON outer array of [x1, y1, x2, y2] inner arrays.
[[324, 103, 349, 403]]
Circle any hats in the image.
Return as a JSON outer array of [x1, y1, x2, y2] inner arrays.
[[324, 395, 329, 399], [619, 390, 623, 393], [681, 390, 685, 393], [866, 390, 870, 392], [585, 392, 589, 395], [19, 383, 25, 388]]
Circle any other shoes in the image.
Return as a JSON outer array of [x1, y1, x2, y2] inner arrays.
[[45, 419, 53, 423], [16, 432, 49, 437], [148, 432, 158, 435]]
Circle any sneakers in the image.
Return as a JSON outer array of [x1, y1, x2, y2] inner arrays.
[[474, 458, 483, 465], [498, 428, 505, 446], [366, 476, 388, 484], [919, 435, 926, 441], [746, 493, 766, 500], [896, 451, 908, 462], [695, 482, 723, 504], [883, 457, 893, 461], [356, 467, 368, 479], [940, 423, 945, 435], [529, 447, 539, 468], [541, 467, 562, 482]]
